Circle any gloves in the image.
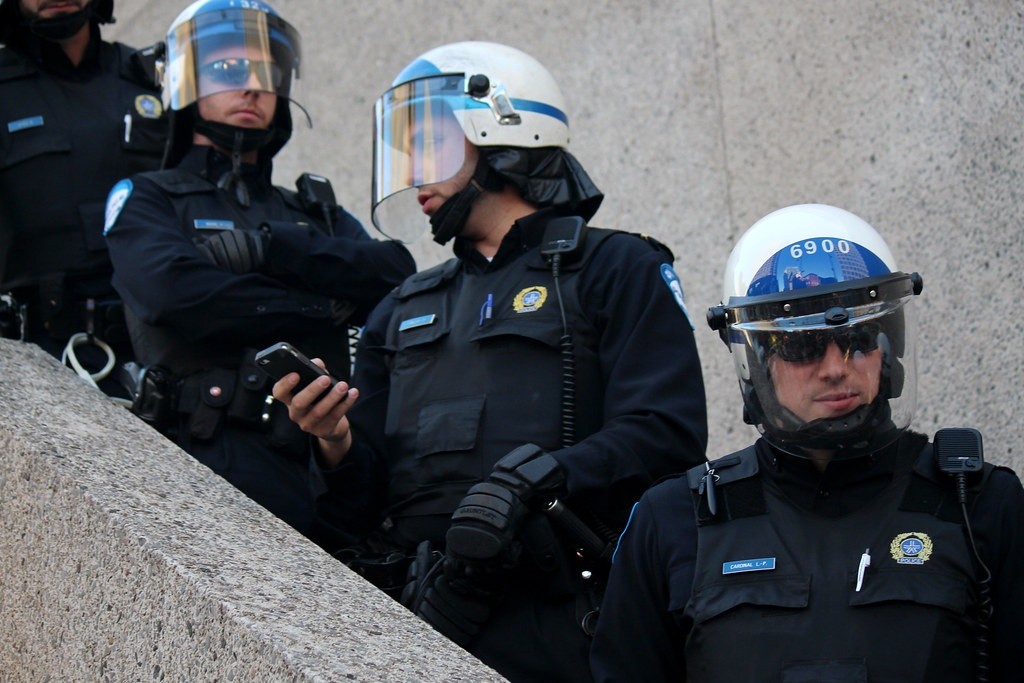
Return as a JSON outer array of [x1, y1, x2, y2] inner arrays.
[[197, 229, 254, 269], [439, 483, 527, 590]]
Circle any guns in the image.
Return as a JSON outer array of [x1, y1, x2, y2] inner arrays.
[[121, 362, 167, 424]]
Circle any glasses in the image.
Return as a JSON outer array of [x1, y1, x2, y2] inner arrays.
[[196, 57, 281, 91], [762, 323, 886, 363]]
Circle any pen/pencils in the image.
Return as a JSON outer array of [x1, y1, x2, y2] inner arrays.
[[854, 548, 872, 592], [479, 293, 493, 327], [124, 109, 134, 142]]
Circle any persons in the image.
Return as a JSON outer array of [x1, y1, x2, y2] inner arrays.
[[102, 0, 418, 559], [1, 0, 185, 401], [587, 203, 1024, 683], [783, 322, 892, 396], [271, 40, 711, 683]]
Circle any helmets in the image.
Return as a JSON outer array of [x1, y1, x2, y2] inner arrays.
[[706, 203, 924, 465], [370, 41, 569, 245], [162, 0, 315, 162]]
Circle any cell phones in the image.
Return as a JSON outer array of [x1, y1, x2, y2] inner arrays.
[[253, 341, 349, 406]]
